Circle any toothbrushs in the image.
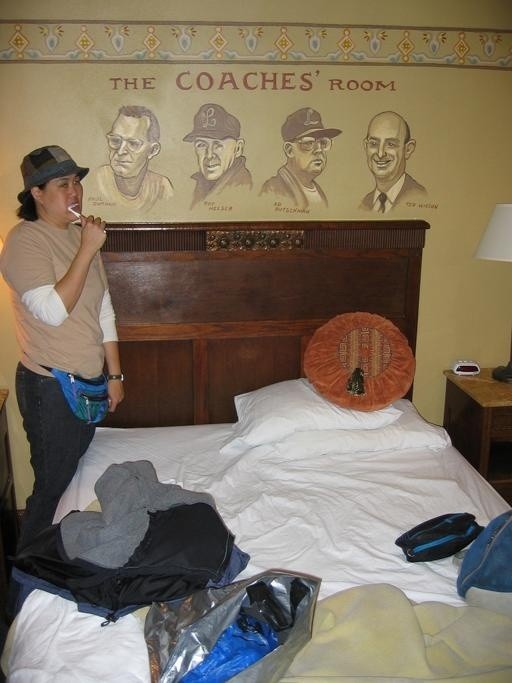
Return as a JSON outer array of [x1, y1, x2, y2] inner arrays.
[[68, 205, 106, 234]]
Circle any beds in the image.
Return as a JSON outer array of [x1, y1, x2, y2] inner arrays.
[[0, 220, 512, 682]]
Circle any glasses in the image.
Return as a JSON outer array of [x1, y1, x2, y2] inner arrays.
[[295, 136, 332, 151], [106, 132, 143, 152]]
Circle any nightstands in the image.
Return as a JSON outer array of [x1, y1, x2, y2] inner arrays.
[[443, 368, 512, 506], [0, 388, 20, 609]]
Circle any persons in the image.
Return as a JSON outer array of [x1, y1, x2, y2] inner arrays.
[[182, 103, 253, 218], [357, 111, 428, 217], [257, 107, 343, 211], [0, 144, 125, 554], [84, 105, 174, 221]]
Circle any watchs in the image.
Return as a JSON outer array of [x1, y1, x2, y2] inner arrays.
[[108, 374, 124, 382]]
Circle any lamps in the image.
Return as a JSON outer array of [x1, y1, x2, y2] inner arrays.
[[474, 204, 512, 385]]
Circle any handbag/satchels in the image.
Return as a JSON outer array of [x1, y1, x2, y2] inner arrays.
[[395, 510, 512, 616], [144, 570, 321, 683], [51, 368, 110, 423]]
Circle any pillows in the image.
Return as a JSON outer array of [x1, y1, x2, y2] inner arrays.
[[220, 378, 405, 454]]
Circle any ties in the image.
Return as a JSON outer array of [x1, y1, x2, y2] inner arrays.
[[379, 194, 386, 213]]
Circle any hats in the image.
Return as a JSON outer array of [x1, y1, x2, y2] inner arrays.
[[22, 146, 89, 193], [181, 104, 240, 140], [282, 108, 342, 140]]
